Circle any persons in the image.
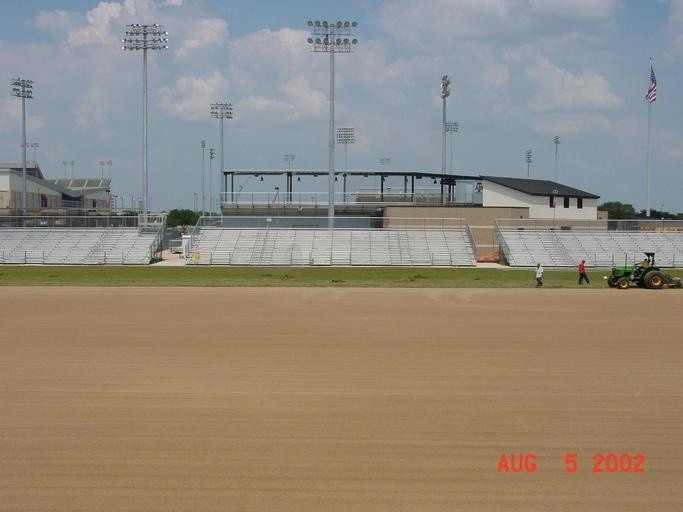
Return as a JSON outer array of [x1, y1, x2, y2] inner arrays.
[[576, 259, 592, 286], [533, 261, 544, 287], [634, 259, 649, 276]]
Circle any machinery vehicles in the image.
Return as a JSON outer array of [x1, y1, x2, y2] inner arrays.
[[608, 252, 682, 289]]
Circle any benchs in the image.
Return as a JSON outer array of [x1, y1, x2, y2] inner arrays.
[[190, 228, 476, 266], [496, 229, 683, 267], [0, 227, 163, 263]]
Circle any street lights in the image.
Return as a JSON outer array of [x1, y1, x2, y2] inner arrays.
[[199, 103, 233, 223], [440, 75, 460, 205], [9, 77, 34, 227], [525, 134, 562, 181], [121, 23, 168, 215], [307, 20, 358, 227]]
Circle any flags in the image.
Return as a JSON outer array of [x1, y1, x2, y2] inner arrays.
[[644, 57, 658, 106]]
